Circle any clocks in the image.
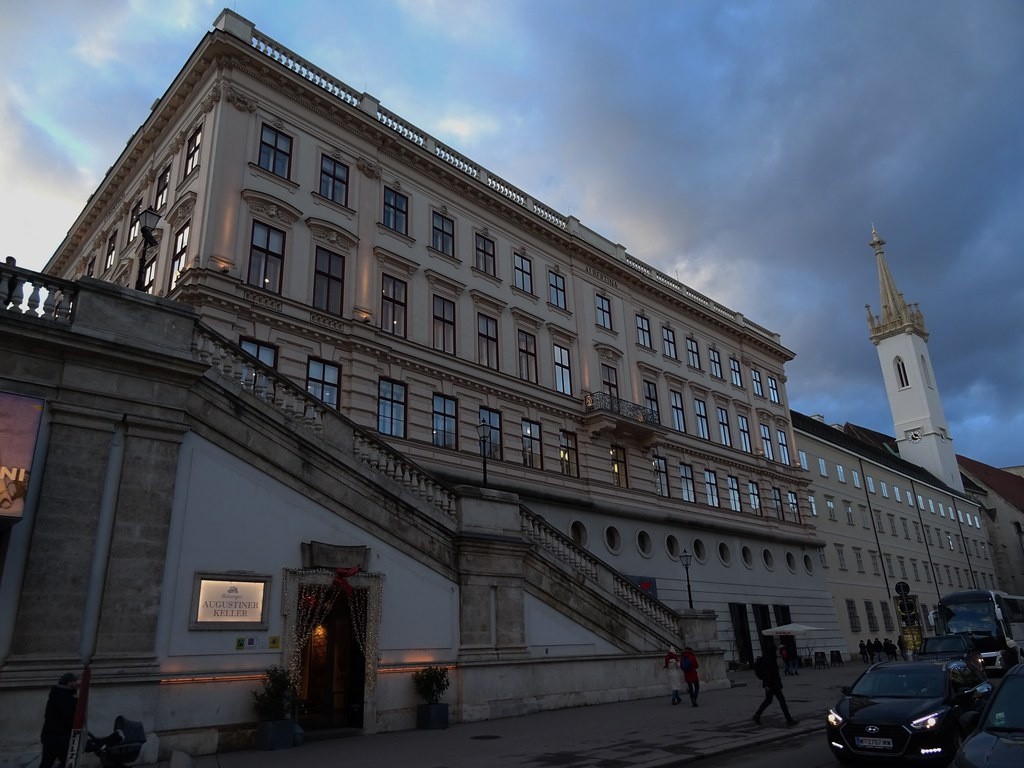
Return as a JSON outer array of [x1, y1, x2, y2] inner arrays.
[[909, 431, 923, 444]]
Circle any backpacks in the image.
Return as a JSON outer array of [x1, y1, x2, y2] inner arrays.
[[754, 656, 766, 680], [680, 656, 691, 673]]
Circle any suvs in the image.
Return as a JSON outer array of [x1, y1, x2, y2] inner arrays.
[[913, 634, 987, 674]]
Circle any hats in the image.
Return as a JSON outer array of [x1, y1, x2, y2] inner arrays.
[[59, 674, 78, 685], [1006, 637, 1017, 648]]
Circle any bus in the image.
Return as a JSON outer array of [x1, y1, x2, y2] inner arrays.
[[927, 588, 1024, 676]]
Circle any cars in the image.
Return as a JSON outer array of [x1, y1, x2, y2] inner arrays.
[[826, 658, 996, 768], [954, 664, 1024, 768]]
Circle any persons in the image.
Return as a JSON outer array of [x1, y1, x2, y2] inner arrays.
[[85, 729, 125, 753], [859, 635, 904, 663], [662, 645, 700, 707], [2, 256, 17, 304], [778, 642, 799, 676], [38, 673, 79, 768], [752, 644, 801, 727]]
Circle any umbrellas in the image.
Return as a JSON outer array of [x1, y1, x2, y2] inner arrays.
[[762, 621, 824, 662]]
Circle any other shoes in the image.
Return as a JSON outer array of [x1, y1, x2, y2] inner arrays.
[[784, 670, 787, 675], [672, 701, 678, 705], [753, 714, 764, 726], [791, 672, 794, 675], [693, 702, 698, 707], [786, 718, 799, 725], [677, 698, 682, 703], [795, 672, 799, 676], [787, 671, 791, 675]]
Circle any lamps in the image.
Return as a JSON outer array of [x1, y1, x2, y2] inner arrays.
[[222, 265, 230, 273], [363, 317, 370, 323]]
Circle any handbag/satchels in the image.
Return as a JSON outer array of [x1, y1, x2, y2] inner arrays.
[[1017, 647, 1024, 663]]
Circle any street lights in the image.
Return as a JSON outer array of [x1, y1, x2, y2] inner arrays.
[[475, 417, 492, 485], [135, 205, 162, 291], [679, 548, 693, 610]]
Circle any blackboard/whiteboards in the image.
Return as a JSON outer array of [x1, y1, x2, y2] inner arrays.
[[814, 652, 828, 666], [830, 650, 842, 663]]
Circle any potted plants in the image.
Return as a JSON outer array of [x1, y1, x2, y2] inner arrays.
[[412, 665, 450, 731], [250, 664, 300, 751]]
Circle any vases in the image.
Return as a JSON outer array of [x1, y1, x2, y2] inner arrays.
[[803, 658, 813, 667], [728, 660, 736, 671]]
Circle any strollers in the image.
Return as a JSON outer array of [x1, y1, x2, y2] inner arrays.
[[85, 715, 147, 768]]
